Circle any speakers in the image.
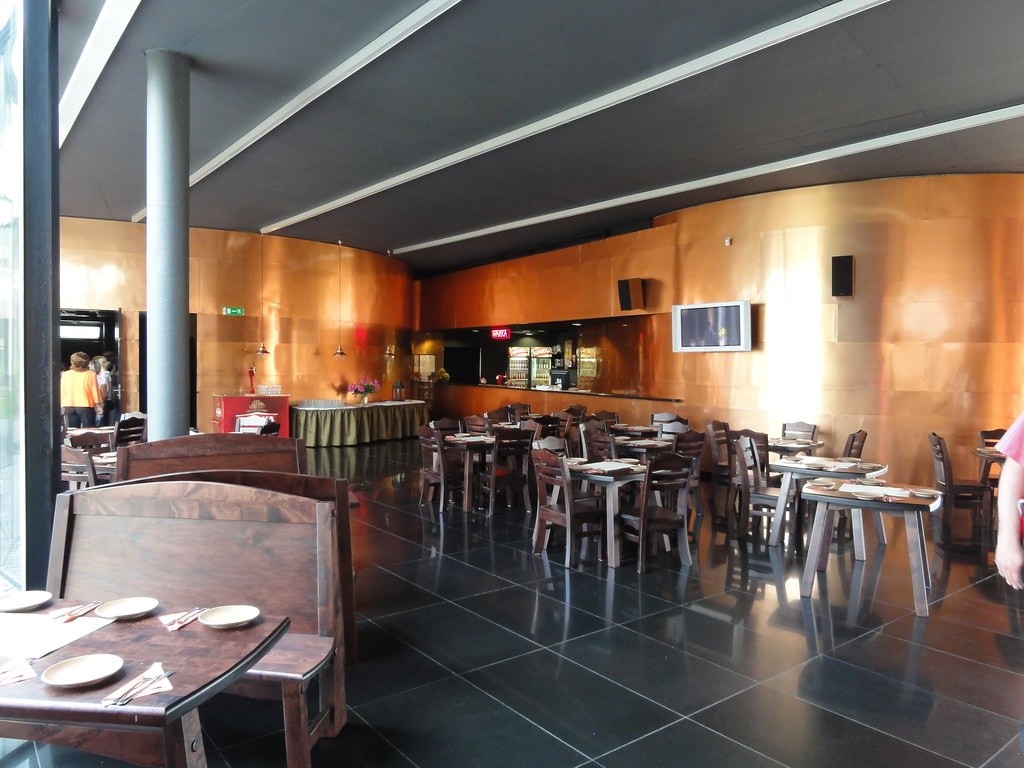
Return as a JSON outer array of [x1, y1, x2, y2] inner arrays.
[[617, 277, 645, 310], [831, 255, 855, 295]]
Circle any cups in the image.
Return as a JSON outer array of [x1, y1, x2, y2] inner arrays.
[[257, 384, 282, 395]]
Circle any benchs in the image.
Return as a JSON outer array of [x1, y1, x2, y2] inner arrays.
[[46, 433, 357, 768]]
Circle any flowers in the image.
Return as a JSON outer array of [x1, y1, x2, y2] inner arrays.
[[348, 376, 382, 398]]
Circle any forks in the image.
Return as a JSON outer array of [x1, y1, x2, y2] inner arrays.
[[175, 608, 206, 624], [102, 662, 162, 707]]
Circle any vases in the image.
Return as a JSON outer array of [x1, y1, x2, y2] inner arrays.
[[361, 395, 368, 405]]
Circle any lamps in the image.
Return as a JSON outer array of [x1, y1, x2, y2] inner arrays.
[[382, 249, 396, 357], [332, 240, 347, 357], [258, 233, 270, 355]]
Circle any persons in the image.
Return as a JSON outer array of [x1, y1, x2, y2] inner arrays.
[[994, 413, 1024, 591], [60, 351, 118, 428]]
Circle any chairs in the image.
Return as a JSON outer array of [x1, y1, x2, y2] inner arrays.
[[416, 402, 867, 575], [59, 408, 147, 490], [929, 432, 992, 568]]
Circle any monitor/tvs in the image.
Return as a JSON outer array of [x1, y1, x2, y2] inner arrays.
[[671, 302, 751, 353]]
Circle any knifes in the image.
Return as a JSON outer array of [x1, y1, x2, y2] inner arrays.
[[163, 607, 199, 627], [117, 670, 175, 706]]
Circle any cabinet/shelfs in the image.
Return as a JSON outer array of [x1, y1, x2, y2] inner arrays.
[[210, 394, 290, 439]]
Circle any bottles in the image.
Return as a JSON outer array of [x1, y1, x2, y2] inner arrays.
[[96, 413, 101, 426], [510, 359, 548, 379]]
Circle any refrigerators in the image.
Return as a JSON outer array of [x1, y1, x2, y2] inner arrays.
[[530, 346, 552, 390], [503, 346, 530, 388]]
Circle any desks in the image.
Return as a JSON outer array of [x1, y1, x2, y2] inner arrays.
[[800, 476, 941, 618], [971, 447, 1006, 565], [0, 599, 291, 768], [979, 428, 1007, 489], [426, 434, 520, 513], [763, 456, 888, 562], [544, 464, 670, 570], [290, 399, 429, 448]]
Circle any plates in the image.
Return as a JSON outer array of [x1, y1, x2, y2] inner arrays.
[[40, 654, 123, 689], [454, 414, 944, 501], [0, 590, 53, 613], [198, 605, 261, 628], [976, 448, 1006, 459], [95, 597, 159, 619]]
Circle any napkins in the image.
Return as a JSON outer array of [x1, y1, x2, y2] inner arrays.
[[49, 604, 85, 620], [158, 608, 209, 632], [102, 661, 173, 708], [0, 657, 38, 686]]
